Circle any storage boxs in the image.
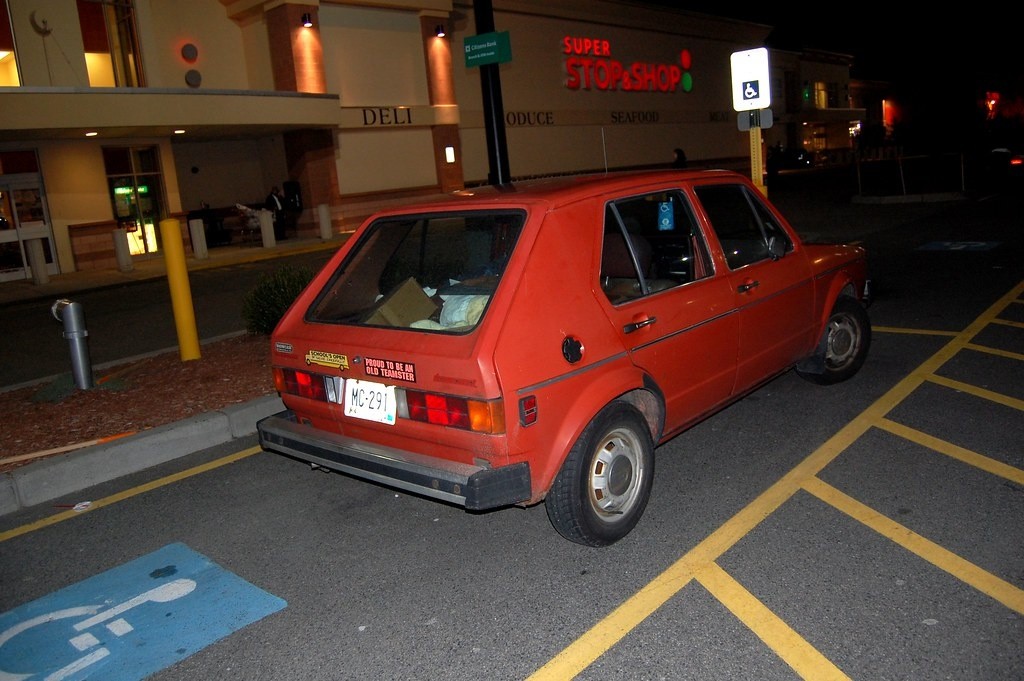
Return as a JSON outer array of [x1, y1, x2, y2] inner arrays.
[[357, 277, 438, 327]]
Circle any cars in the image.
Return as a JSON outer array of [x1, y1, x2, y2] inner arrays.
[[256, 166, 884, 549]]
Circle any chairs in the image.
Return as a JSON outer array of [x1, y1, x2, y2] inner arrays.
[[600, 233, 678, 301], [437, 230, 500, 296]]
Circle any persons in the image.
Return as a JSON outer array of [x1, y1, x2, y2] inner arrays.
[[265, 180, 303, 241], [671, 146, 687, 163], [765, 140, 837, 171]]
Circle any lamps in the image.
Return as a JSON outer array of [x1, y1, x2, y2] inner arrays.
[[434, 24, 446, 38], [301, 12, 312, 28]]
